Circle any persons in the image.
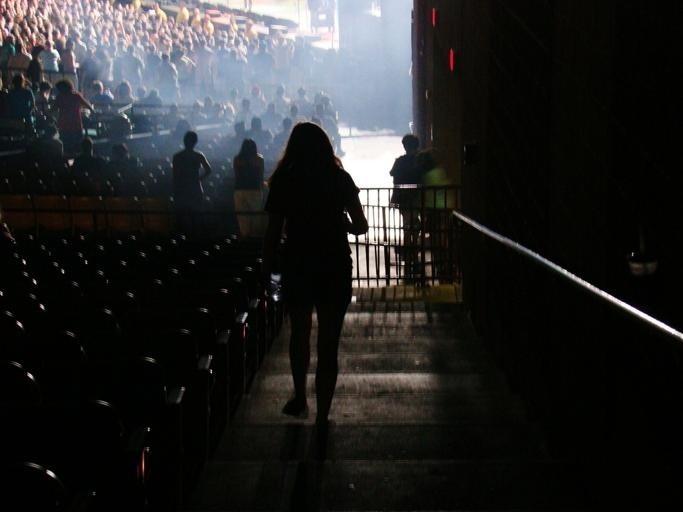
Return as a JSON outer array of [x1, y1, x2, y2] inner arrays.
[[231, 137, 268, 238], [389, 133, 422, 260], [263, 119, 370, 442], [1, 0, 346, 178], [172, 130, 214, 232]]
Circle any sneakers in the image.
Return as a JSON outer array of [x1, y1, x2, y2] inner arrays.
[[280, 398, 340, 435]]
